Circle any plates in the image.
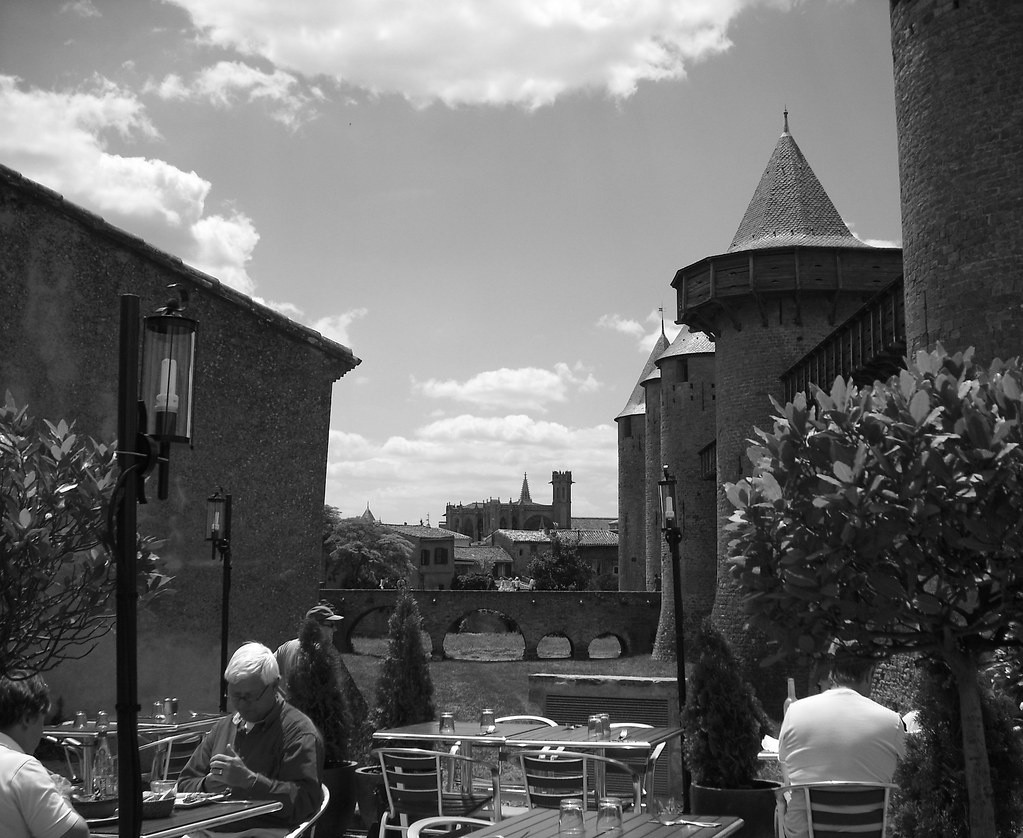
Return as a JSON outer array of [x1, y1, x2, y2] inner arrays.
[[85, 817, 119, 822], [174, 792, 224, 809]]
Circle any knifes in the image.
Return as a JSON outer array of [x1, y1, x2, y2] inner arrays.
[[648, 820, 722, 826]]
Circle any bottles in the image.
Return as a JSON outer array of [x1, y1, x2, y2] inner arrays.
[[783, 677, 798, 716]]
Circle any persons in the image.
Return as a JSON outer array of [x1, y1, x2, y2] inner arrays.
[[488, 574, 536, 591], [379, 577, 405, 589], [273, 606, 345, 699], [777, 647, 905, 838], [0, 666, 90, 838], [177, 643, 323, 838]]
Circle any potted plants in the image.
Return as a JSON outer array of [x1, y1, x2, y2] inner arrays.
[[355, 581, 443, 832], [283, 617, 359, 838], [681, 615, 784, 838]]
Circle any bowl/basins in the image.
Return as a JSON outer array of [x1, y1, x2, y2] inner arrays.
[[72, 794, 117, 819], [143, 796, 176, 820]]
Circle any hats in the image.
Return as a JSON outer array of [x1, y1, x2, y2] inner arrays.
[[306, 606, 344, 620]]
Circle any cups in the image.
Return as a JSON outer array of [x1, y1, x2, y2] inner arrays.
[[72, 711, 87, 729], [439, 711, 455, 735], [480, 709, 496, 734], [587, 715, 604, 742], [149, 779, 179, 796], [151, 703, 163, 719], [557, 798, 585, 838], [164, 701, 177, 725], [596, 714, 611, 742], [95, 711, 109, 728], [595, 799, 624, 838]]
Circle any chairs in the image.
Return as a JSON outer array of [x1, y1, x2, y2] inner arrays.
[[44, 716, 899, 838]]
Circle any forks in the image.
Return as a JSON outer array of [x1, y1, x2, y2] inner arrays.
[[652, 813, 717, 828]]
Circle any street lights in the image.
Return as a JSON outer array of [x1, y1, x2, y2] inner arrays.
[[105, 280, 206, 838], [204, 483, 234, 715], [656, 462, 693, 815]]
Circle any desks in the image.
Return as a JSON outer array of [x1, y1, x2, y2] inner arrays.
[[504, 725, 684, 811], [372, 721, 549, 796], [42, 712, 232, 795], [462, 806, 745, 837], [87, 798, 284, 838]]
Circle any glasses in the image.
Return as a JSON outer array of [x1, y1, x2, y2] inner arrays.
[[321, 622, 334, 628], [224, 682, 271, 704]]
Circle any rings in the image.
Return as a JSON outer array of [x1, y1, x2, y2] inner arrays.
[[220, 769, 223, 774]]
